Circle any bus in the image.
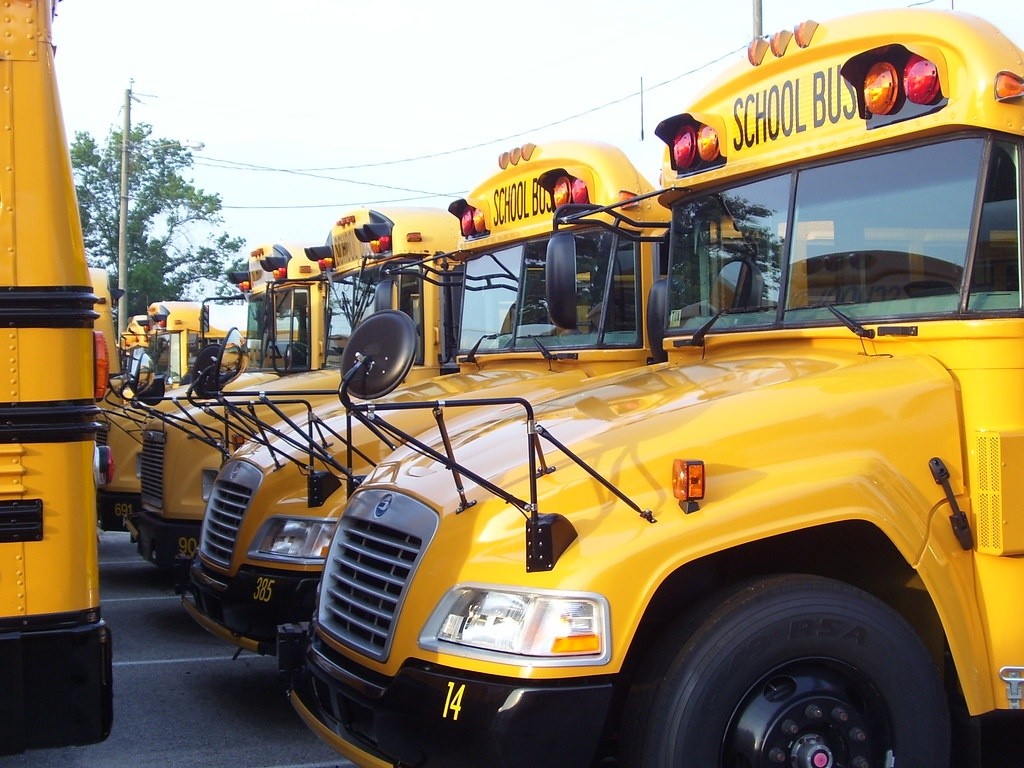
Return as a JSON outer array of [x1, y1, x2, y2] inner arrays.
[[2, 0, 122, 752], [102, 137, 1022, 672], [268, 5, 1022, 767]]
